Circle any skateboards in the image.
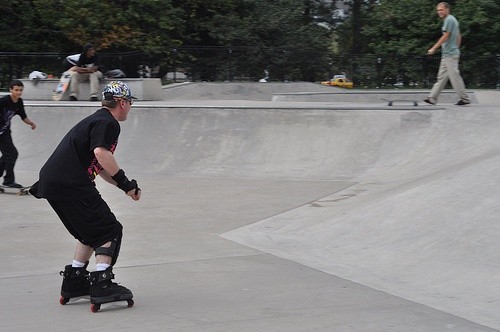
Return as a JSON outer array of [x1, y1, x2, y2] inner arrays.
[[0, 184, 32, 196], [379, 97, 430, 106], [52, 70, 73, 101]]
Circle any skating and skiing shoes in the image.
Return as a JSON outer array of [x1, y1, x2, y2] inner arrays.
[[90, 265, 134, 313], [60, 260, 91, 305]]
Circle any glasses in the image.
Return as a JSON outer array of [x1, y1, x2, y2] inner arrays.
[[124, 100, 134, 105]]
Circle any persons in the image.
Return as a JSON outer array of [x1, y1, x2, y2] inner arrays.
[[0, 80, 36, 188], [68, 43, 104, 101], [422, 1, 472, 106], [25, 80, 142, 304]]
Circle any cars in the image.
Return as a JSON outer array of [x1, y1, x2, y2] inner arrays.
[[164, 72, 189, 84], [321, 75, 354, 89]]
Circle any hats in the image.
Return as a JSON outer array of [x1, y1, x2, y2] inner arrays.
[[101, 81, 138, 100], [83, 43, 93, 50]]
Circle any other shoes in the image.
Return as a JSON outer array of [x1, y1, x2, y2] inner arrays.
[[424, 100, 436, 105], [91, 97, 97, 101], [70, 96, 77, 101], [454, 100, 469, 105]]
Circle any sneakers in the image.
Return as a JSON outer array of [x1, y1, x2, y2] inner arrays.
[[3, 182, 22, 188]]
